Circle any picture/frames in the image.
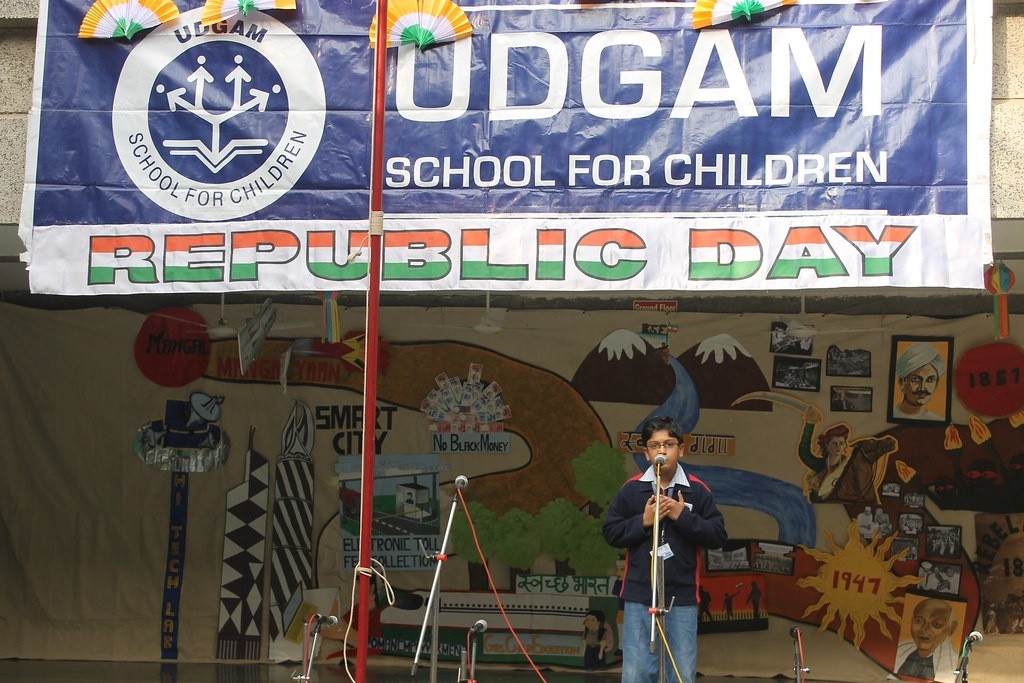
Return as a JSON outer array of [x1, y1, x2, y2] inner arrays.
[[887, 334, 955, 426], [771, 355, 822, 392]]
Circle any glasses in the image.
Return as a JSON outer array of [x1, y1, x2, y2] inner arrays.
[[646, 441, 680, 449]]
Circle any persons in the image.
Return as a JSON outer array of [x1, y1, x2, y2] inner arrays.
[[601, 414, 730, 683]]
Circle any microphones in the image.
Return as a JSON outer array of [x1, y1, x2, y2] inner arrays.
[[315, 614, 339, 627], [966, 631, 983, 644], [654, 454, 667, 466], [469, 620, 488, 635], [790, 626, 803, 638], [454, 475, 469, 488]]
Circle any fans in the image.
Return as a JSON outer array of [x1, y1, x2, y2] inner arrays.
[[400, 290, 557, 335], [732, 296, 894, 339], [153, 293, 316, 340]]
